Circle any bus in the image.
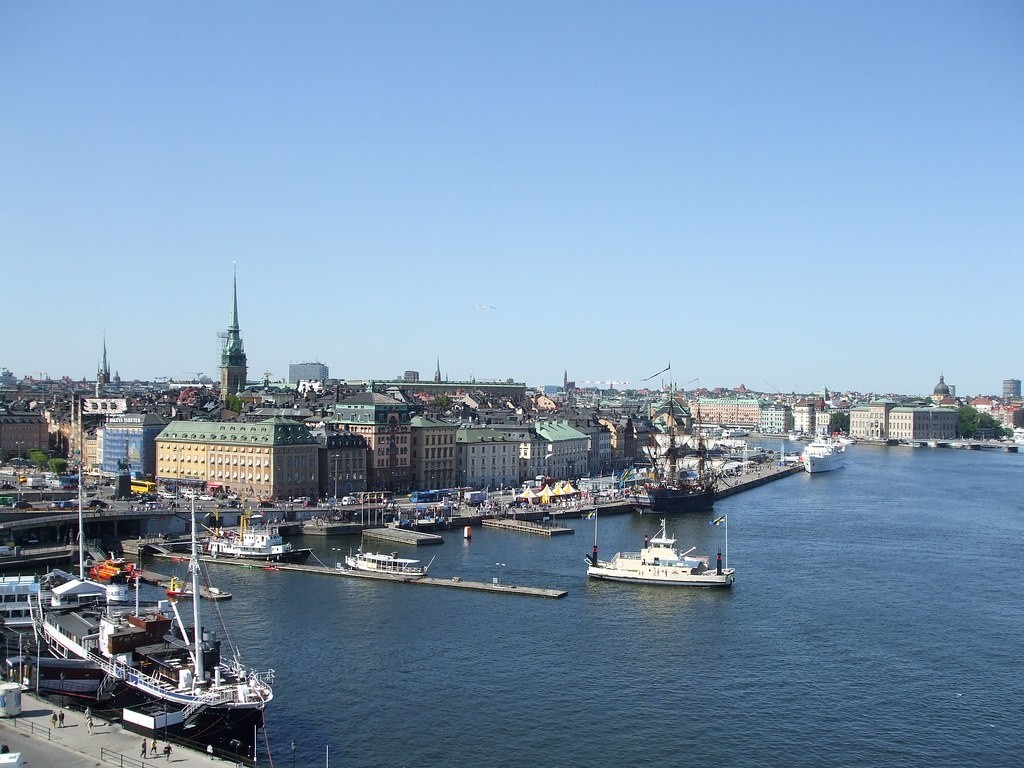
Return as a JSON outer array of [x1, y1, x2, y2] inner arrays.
[[293, 487, 472, 508], [130, 479, 157, 495]]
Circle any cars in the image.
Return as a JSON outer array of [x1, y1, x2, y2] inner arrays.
[[50, 502, 60, 507], [259, 500, 274, 508], [17, 475, 27, 482], [201, 495, 215, 501], [59, 501, 79, 509], [12, 500, 32, 509], [87, 500, 108, 508]]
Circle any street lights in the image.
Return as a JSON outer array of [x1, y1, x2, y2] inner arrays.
[[15, 440, 24, 502]]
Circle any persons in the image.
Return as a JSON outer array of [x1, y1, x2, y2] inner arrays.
[[718, 463, 778, 488], [207, 745, 213, 760], [150, 738, 157, 755], [163, 743, 172, 761], [0, 745, 9, 754], [49, 706, 93, 734], [399, 493, 590, 516], [139, 739, 147, 758]]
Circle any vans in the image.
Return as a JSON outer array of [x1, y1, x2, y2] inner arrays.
[[0, 497, 14, 507]]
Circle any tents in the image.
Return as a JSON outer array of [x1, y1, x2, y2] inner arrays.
[[516, 482, 581, 498]]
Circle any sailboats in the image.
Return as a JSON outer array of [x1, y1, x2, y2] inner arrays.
[[642, 361, 729, 513]]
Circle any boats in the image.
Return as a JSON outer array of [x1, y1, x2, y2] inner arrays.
[[722, 428, 749, 438], [789, 434, 802, 442], [583, 506, 736, 588], [800, 436, 845, 473], [187, 517, 315, 564], [343, 551, 436, 578], [840, 438, 856, 445], [0, 469, 275, 736]]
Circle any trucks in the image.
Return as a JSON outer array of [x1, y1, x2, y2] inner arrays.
[[184, 492, 199, 500]]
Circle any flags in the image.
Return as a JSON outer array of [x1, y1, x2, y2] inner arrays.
[[709, 515, 726, 527], [581, 509, 597, 521]]
